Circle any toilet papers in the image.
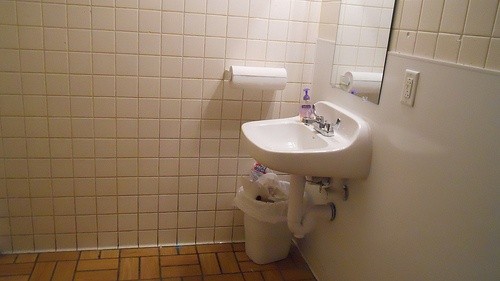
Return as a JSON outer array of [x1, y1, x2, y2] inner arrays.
[[227, 64, 287, 91], [343, 71, 385, 94]]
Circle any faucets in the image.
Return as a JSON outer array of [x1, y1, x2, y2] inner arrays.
[[301, 115, 326, 128]]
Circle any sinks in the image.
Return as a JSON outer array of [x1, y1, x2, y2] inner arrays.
[[241, 100, 373, 181]]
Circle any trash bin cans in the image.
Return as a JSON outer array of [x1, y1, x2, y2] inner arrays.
[[237, 180, 293, 265]]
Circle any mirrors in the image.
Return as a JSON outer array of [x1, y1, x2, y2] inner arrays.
[[330, 0, 395, 105]]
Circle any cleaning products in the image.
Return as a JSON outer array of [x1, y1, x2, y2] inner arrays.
[[299, 88, 314, 118]]
